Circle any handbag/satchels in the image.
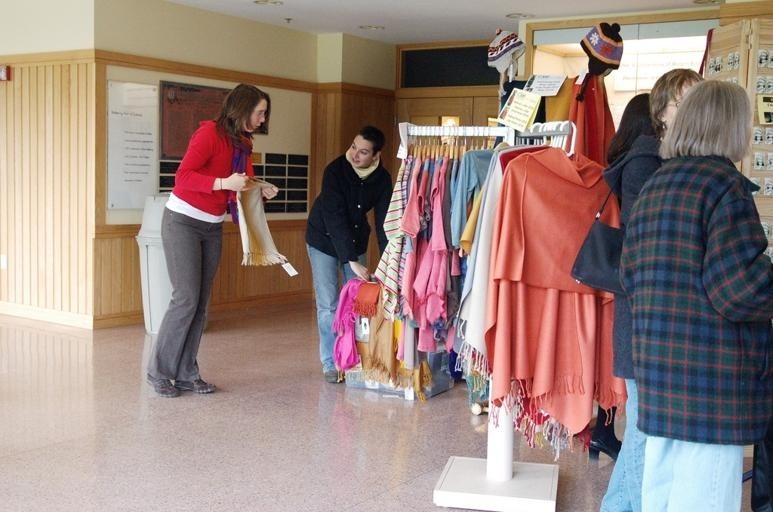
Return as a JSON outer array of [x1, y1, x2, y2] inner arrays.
[[572, 157, 635, 297]]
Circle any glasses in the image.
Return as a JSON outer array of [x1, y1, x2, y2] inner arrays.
[[665, 102, 681, 107]]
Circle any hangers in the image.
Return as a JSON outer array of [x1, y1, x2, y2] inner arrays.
[[399, 121, 578, 163]]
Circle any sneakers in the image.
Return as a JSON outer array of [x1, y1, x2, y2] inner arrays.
[[177, 376, 218, 396], [144, 374, 177, 395], [323, 369, 342, 381]]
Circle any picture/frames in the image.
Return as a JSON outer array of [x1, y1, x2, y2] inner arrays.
[[157, 79, 232, 160]]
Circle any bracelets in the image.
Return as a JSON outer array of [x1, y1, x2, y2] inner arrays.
[[220, 178, 222, 190]]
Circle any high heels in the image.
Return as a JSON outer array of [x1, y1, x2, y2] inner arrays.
[[589, 428, 625, 463]]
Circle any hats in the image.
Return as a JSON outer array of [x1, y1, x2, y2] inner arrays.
[[576, 18, 624, 103], [486, 27, 527, 98]]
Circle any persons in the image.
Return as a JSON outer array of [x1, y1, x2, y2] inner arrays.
[[587, 94, 653, 464], [601, 69, 711, 512], [618, 78, 773, 512], [145, 83, 279, 397], [305, 125, 394, 384]]
